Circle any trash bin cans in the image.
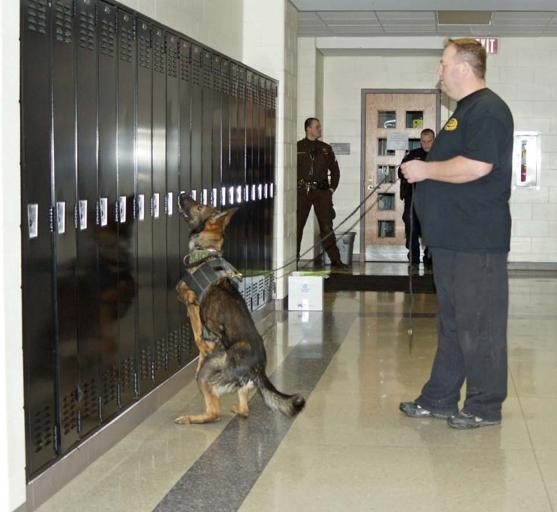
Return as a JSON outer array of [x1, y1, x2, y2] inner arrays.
[[323, 232, 356, 265]]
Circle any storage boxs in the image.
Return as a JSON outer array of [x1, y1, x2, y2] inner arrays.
[[287, 270, 331, 311]]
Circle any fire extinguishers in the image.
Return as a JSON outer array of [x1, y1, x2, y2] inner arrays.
[[521, 141, 527, 181]]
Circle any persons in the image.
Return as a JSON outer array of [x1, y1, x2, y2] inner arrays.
[[297, 118, 349, 271], [398, 129, 435, 274], [399, 38, 514, 430]]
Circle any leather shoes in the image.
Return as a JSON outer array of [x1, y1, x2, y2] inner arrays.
[[448, 408, 502, 429], [331, 262, 347, 268], [400, 401, 458, 419], [408, 264, 418, 270]]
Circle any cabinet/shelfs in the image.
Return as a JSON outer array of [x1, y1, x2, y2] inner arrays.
[[20, 0, 278, 483]]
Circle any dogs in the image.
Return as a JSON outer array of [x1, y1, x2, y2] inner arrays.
[[175, 191, 306, 426]]
